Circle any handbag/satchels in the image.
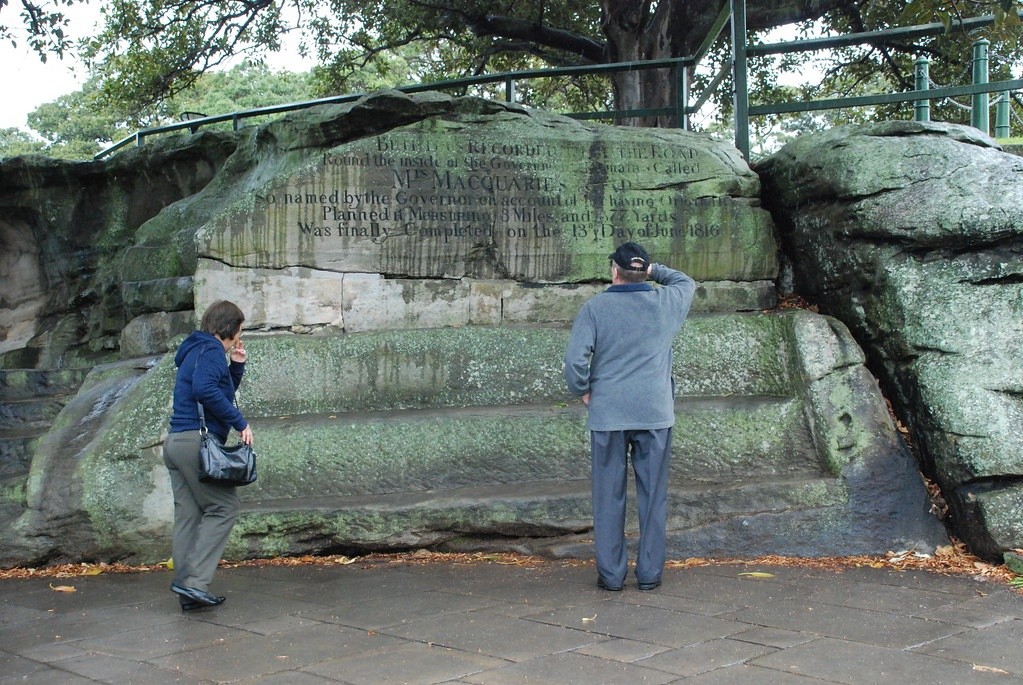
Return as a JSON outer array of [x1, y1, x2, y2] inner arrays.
[[194, 344, 258, 486]]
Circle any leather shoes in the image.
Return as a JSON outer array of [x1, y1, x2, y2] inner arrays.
[[181, 596, 225, 612], [170, 581, 218, 605]]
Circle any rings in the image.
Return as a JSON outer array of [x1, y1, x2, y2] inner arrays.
[[244, 350, 246, 353]]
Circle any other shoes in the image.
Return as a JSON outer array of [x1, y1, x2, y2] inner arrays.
[[597, 575, 623, 592], [639, 579, 661, 590]]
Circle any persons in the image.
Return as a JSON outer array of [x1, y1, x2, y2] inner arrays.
[[565, 242, 696, 591], [163, 300, 254, 611]]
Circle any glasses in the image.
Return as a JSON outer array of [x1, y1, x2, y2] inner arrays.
[[231, 328, 245, 339]]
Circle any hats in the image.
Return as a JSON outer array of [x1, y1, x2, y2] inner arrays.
[[608, 242, 649, 271]]
